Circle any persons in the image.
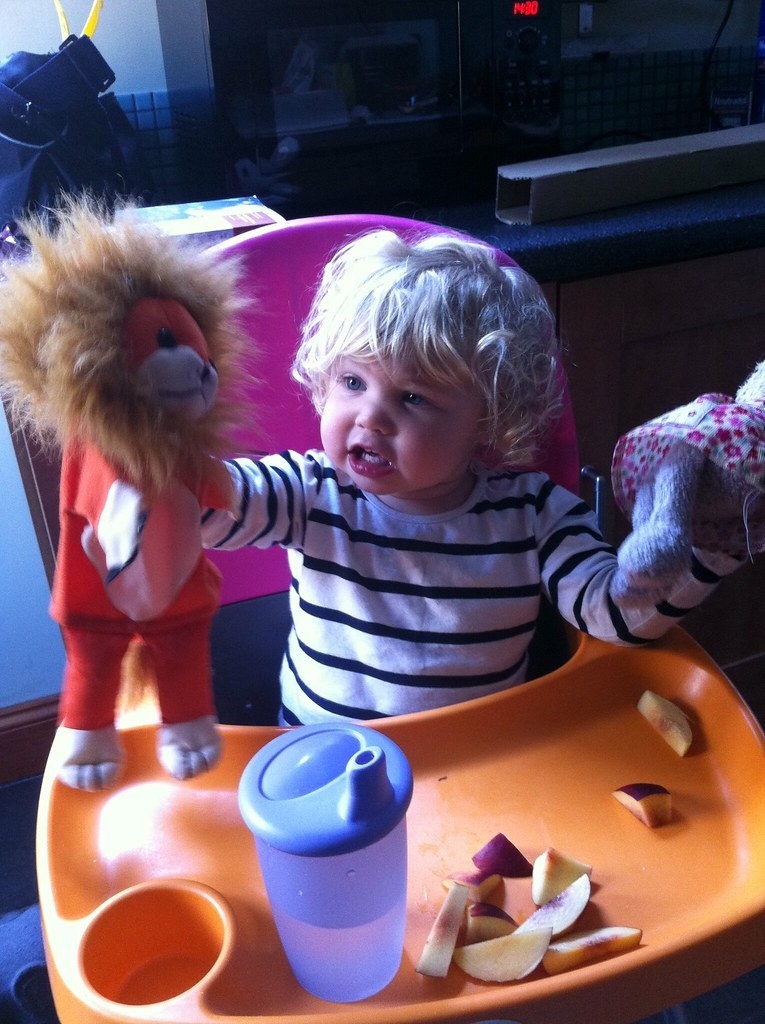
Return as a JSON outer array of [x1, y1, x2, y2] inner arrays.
[[201, 232, 748, 736]]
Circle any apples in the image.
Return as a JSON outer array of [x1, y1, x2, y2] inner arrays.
[[636, 689, 694, 757], [610, 782, 672, 829], [414, 832, 645, 984]]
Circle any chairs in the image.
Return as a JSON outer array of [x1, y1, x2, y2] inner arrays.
[[198, 211, 583, 597]]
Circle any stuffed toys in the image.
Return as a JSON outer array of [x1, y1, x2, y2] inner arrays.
[[610, 362, 765, 612], [0, 193, 261, 793]]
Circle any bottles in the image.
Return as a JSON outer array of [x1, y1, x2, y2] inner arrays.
[[238, 721, 414, 1003]]
